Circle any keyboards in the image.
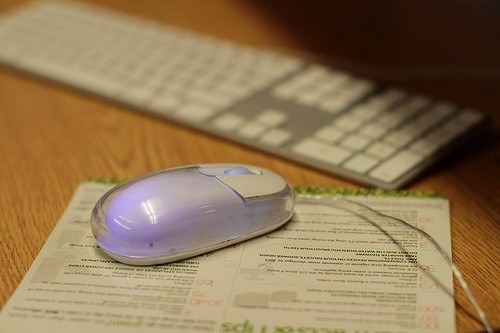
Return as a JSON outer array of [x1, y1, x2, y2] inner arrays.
[[0, 4, 488, 194]]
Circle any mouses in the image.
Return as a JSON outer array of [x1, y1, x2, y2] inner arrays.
[[89, 163, 294, 268]]
